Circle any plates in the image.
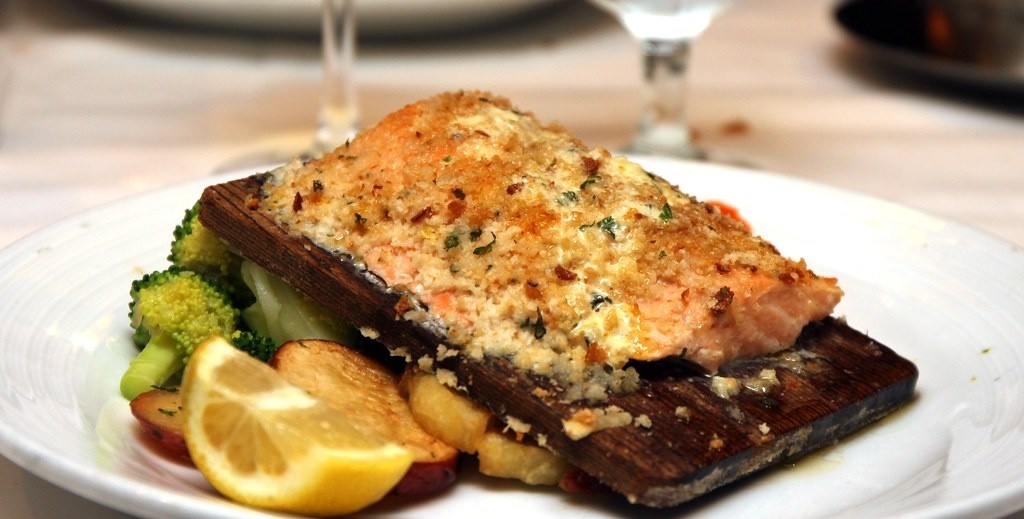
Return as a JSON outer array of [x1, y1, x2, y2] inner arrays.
[[2, 155, 1024, 519], [837, 2, 1023, 95]]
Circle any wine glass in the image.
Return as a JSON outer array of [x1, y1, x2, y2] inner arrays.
[[315, 0, 360, 149], [598, 2, 729, 160]]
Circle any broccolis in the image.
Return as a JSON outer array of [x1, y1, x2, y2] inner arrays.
[[120, 201, 277, 397]]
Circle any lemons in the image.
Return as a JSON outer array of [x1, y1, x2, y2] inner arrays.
[[178, 337, 413, 516]]
